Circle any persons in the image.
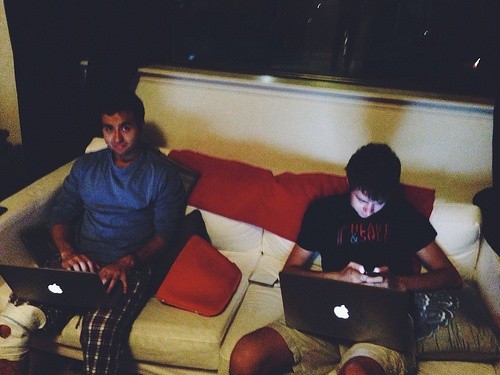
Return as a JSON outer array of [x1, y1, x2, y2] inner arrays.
[[0, 88, 190, 375], [228, 142, 464, 375]]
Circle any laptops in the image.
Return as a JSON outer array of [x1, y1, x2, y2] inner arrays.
[[278, 270, 413, 355], [0, 264, 124, 310]]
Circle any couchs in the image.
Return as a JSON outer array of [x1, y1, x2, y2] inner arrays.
[[0, 137, 500, 375]]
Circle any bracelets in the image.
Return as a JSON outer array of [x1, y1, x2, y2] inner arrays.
[[124, 249, 139, 267]]
